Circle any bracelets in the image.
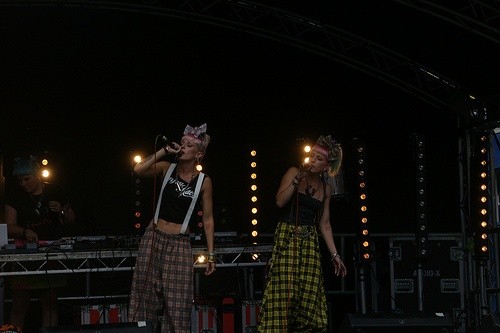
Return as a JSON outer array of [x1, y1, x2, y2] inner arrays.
[[291, 175, 302, 187], [330, 253, 340, 262], [207, 253, 215, 263], [163, 146, 169, 154]]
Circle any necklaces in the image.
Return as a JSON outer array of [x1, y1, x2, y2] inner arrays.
[[305, 175, 321, 200], [175, 163, 194, 193]]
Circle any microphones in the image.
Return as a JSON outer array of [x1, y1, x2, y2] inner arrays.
[[300, 163, 312, 168], [162, 135, 177, 148]]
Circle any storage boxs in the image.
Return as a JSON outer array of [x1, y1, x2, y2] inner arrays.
[[80, 298, 263, 333]]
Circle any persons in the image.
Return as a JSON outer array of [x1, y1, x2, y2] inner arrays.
[[127, 122, 216, 333], [0, 158, 75, 333], [255, 135, 347, 333]]
